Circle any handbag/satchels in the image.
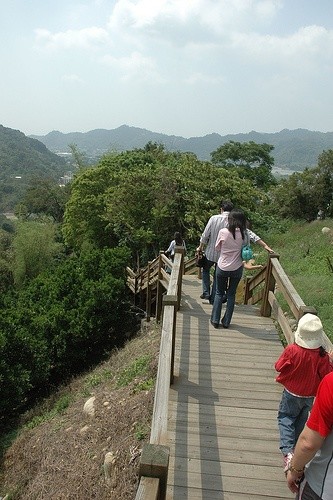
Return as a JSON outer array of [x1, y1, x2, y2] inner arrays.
[[195, 248, 207, 266], [242, 243, 254, 261]]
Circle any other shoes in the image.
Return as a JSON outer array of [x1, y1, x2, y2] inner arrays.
[[210, 319, 219, 329], [283, 450, 294, 471], [221, 319, 228, 328], [200, 293, 209, 300]]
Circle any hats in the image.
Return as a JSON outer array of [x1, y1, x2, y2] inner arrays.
[[295, 313, 324, 350]]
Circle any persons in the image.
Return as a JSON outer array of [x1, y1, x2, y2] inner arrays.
[[274, 313, 333, 473], [210, 209, 249, 329], [286, 372, 333, 500], [165, 232, 187, 261], [196, 201, 275, 305]]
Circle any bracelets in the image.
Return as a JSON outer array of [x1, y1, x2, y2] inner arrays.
[[288, 460, 305, 473]]
